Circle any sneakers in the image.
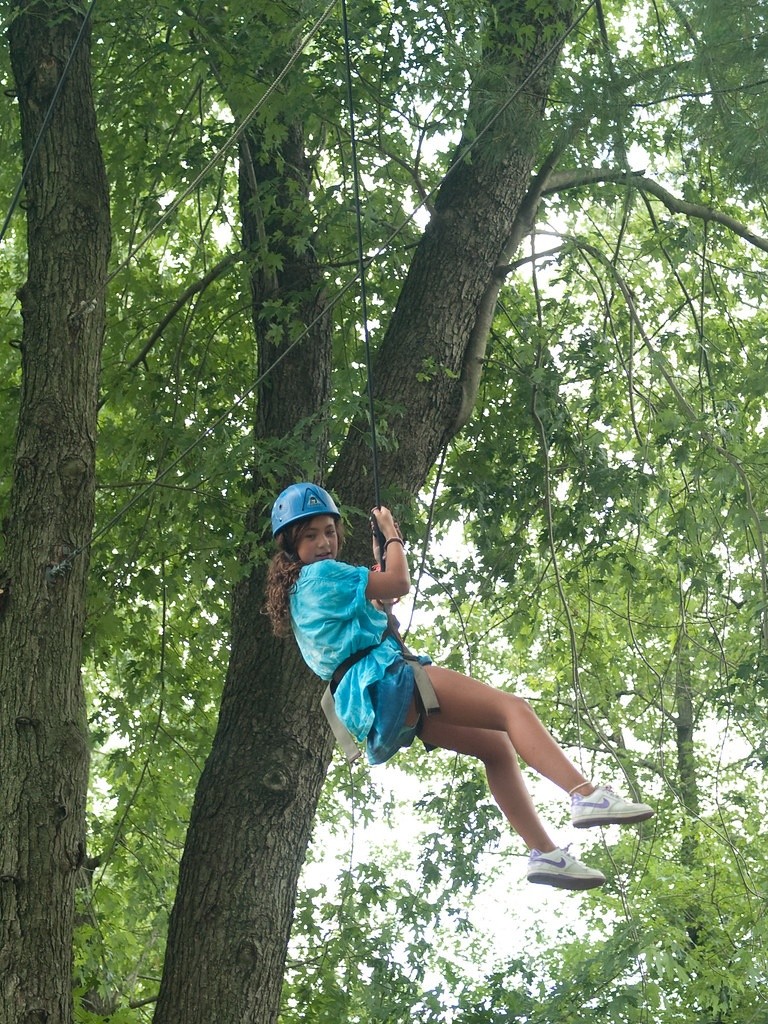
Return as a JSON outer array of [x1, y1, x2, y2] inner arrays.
[[528, 848, 606, 890], [572, 787, 654, 829]]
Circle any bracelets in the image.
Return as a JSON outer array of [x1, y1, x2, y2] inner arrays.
[[381, 537, 404, 559]]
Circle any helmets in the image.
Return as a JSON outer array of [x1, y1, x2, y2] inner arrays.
[[271, 483, 340, 540]]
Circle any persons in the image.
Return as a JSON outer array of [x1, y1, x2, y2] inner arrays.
[[260, 483, 654, 890]]
